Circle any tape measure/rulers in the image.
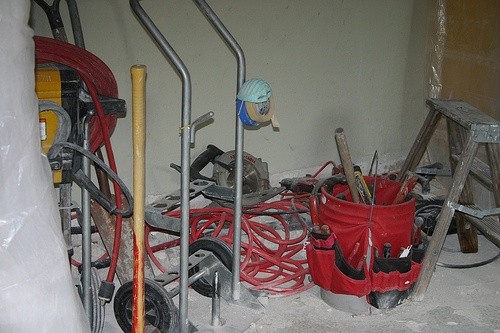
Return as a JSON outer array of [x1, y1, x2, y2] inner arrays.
[[415, 167, 452, 176]]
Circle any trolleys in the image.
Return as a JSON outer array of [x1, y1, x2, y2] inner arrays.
[[111, 0, 245, 332]]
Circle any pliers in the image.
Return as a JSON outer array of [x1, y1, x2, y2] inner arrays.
[[412, 224, 420, 248]]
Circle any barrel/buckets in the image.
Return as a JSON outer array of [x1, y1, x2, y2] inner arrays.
[[320, 177, 415, 313]]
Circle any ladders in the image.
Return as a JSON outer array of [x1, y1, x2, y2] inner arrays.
[[389, 97, 500, 303]]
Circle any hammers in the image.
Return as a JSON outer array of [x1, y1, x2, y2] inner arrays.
[[390, 171, 431, 205]]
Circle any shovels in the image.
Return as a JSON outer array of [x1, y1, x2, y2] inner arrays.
[[36, 1, 157, 288]]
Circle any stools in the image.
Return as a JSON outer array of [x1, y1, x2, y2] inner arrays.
[[384, 98, 500, 302]]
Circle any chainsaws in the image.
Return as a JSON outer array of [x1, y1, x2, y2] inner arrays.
[[187, 144, 271, 203], [261, 177, 320, 205]]
